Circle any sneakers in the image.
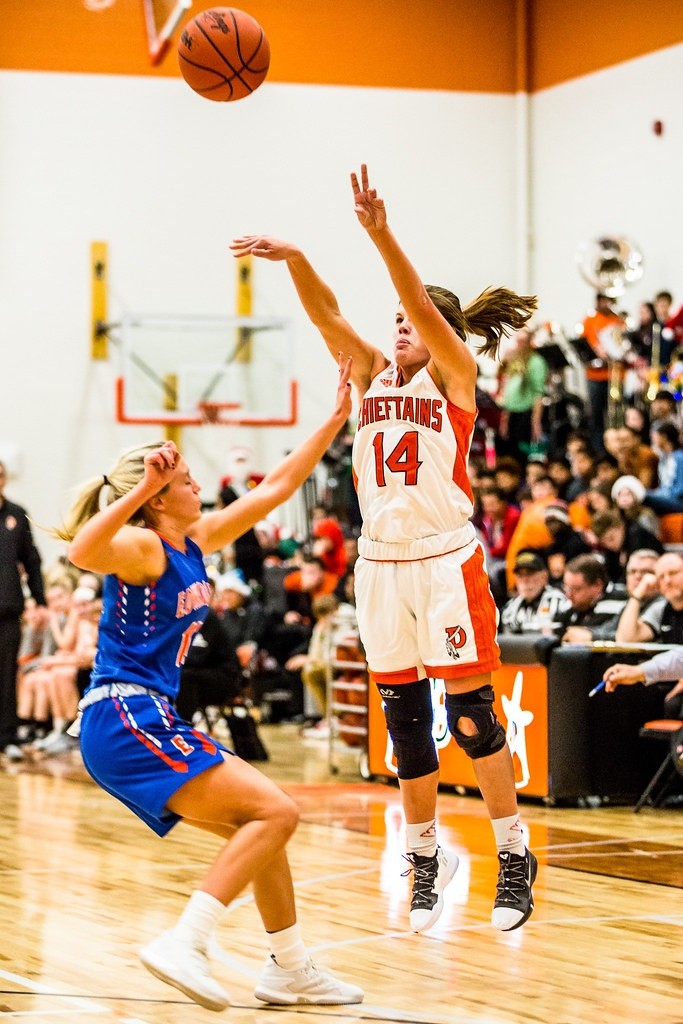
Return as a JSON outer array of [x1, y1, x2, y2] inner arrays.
[[491, 845, 537, 933], [399, 844, 459, 934], [137, 929, 229, 1013], [254, 952, 364, 1005]]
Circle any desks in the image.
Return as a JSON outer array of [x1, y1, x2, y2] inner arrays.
[[368, 635, 683, 809]]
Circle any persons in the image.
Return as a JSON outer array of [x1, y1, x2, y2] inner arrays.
[[67, 351, 364, 1013], [1, 293, 683, 761], [230, 164, 538, 934]]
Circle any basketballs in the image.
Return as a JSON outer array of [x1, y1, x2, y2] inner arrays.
[[177, 5, 272, 103]]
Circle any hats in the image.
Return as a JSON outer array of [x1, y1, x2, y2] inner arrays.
[[610, 474, 648, 504], [513, 552, 546, 571]]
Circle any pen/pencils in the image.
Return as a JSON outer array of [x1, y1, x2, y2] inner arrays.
[[588, 681, 605, 697]]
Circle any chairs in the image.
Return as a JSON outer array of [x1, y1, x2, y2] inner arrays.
[[635, 718, 683, 810]]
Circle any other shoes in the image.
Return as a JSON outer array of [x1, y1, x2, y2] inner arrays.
[[5, 744, 28, 763]]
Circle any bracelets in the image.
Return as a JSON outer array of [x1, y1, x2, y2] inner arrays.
[[630, 594, 643, 602]]
